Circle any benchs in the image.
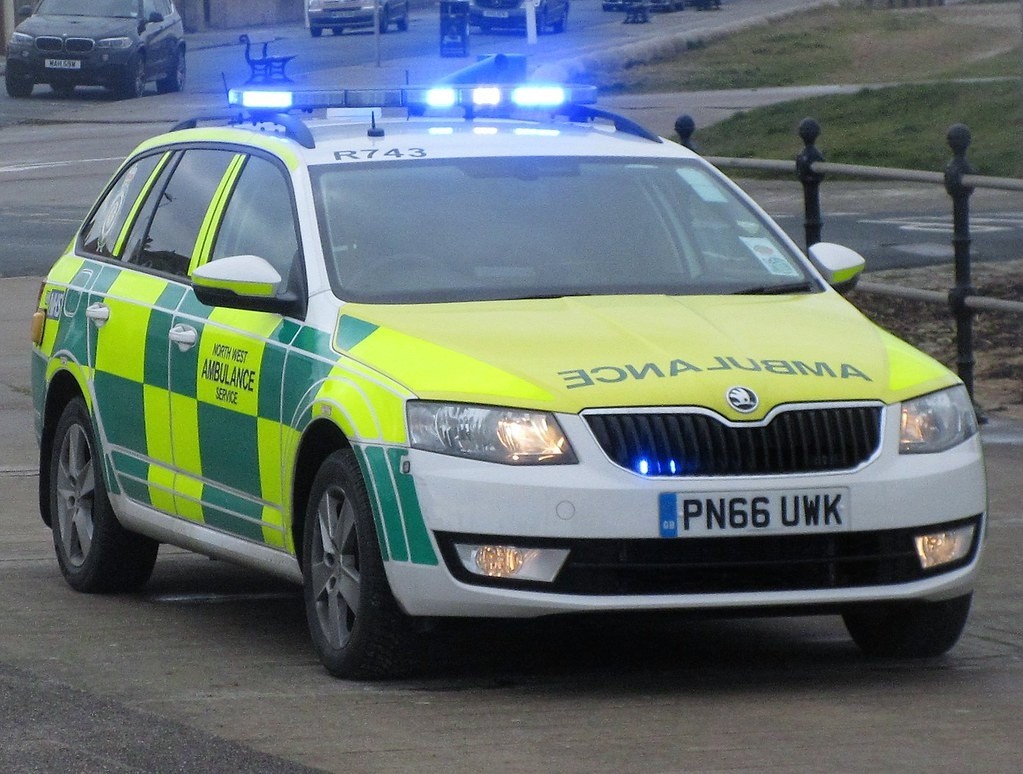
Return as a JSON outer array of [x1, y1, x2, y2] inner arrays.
[[240, 33, 295, 85], [620, 0, 651, 24], [697, 0, 720, 11]]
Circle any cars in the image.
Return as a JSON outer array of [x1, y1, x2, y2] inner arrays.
[[602, 0, 724, 14], [5, 0, 187, 100], [305, 0, 410, 33], [467, 0, 572, 37]]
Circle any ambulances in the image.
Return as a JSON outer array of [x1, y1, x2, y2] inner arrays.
[[29, 78, 986, 685]]
